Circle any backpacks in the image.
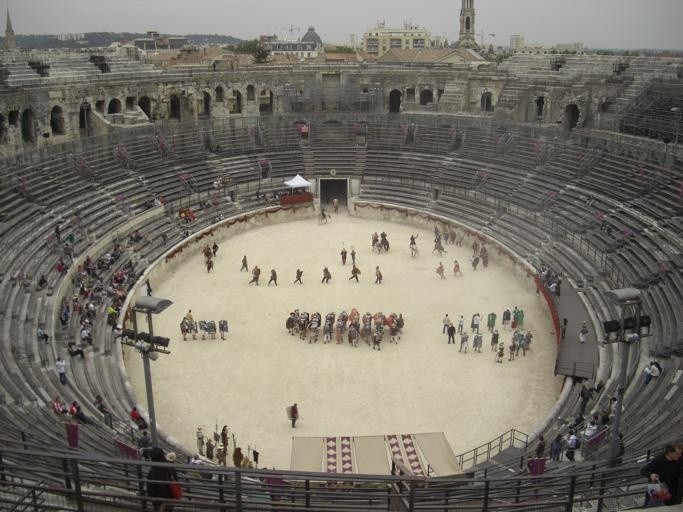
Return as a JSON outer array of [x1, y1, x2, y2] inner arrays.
[[575, 439, 581, 449]]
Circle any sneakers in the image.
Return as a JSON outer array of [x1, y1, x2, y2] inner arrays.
[[448, 342, 455, 344], [288, 331, 403, 351], [442, 323, 524, 334], [459, 348, 529, 363], [183, 337, 226, 341]]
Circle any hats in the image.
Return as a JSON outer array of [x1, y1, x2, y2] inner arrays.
[[218, 443, 224, 449], [166, 452, 176, 461], [207, 438, 213, 442]]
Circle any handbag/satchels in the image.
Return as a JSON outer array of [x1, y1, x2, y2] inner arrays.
[[169, 480, 182, 500], [647, 478, 671, 501]]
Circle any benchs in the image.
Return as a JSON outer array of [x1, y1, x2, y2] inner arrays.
[[1, 120, 683, 223], [216, 464, 457, 511], [0, 222, 217, 510], [457, 223, 683, 510]]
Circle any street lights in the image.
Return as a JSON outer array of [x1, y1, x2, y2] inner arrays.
[[595, 286, 653, 486], [119, 295, 175, 448]]
